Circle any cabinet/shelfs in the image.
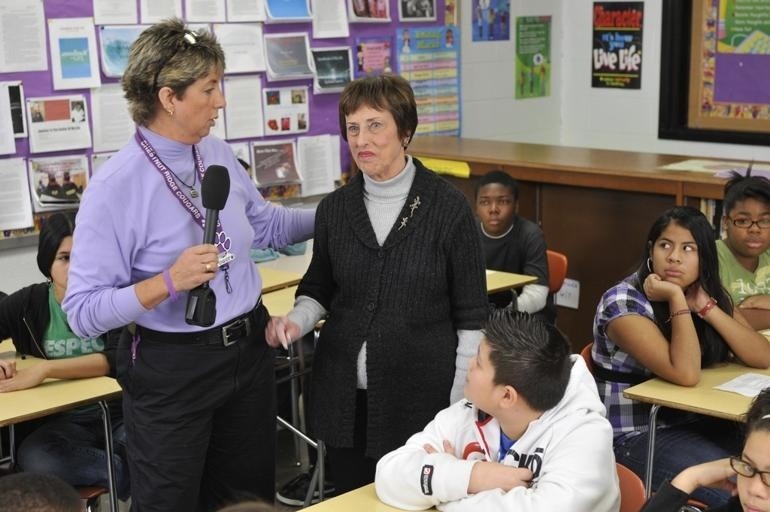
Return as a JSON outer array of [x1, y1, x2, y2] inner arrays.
[[400, 134, 770, 321]]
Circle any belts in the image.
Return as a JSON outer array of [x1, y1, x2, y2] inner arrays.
[[135, 302, 271, 348]]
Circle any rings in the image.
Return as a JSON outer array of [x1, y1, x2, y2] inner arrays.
[[206, 263, 212, 271]]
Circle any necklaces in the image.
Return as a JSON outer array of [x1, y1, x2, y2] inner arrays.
[[134, 127, 201, 200]]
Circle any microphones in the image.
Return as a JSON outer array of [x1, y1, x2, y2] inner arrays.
[[184, 166, 230, 326]]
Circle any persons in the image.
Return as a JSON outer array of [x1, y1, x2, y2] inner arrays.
[[468, 169, 556, 326], [640, 385, 770, 510], [60, 18, 316, 512], [712, 171, 770, 336], [585, 202, 770, 508], [263, 72, 494, 493], [36, 172, 85, 200], [71, 102, 86, 123], [31, 108, 44, 123], [372, 306, 626, 510], [0, 210, 131, 502], [402, 0, 434, 18]]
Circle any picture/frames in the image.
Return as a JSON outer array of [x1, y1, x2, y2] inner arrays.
[[657, 1, 770, 149]]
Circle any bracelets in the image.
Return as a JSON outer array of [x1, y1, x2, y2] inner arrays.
[[162, 268, 177, 298], [668, 306, 692, 318], [696, 296, 719, 320]]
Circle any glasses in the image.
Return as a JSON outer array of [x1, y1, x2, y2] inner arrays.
[[730, 454, 769, 484], [727, 216, 770, 229], [152, 29, 203, 93]]
